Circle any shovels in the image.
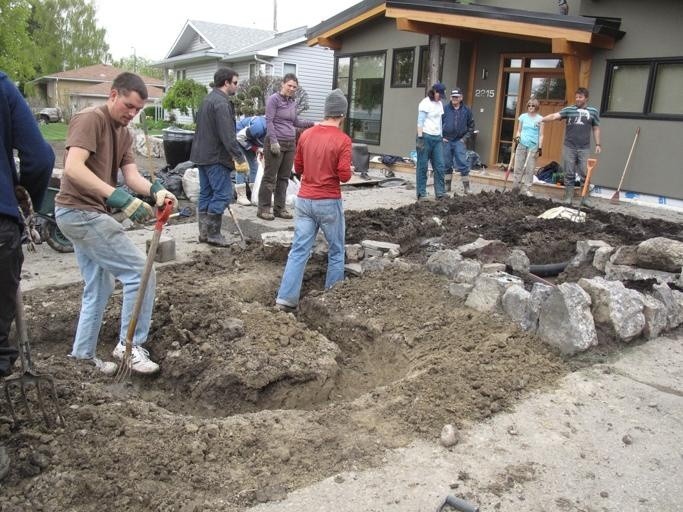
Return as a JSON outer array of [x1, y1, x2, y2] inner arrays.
[[511, 150, 531, 195], [227, 205, 251, 250]]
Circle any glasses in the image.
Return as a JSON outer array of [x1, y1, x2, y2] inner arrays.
[[527, 104, 536, 108]]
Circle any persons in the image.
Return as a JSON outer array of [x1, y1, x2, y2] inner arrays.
[[233, 116, 266, 206], [441, 87, 475, 194], [54, 71, 178, 376], [513, 98, 545, 198], [275, 88, 355, 315], [537, 88, 602, 204], [190, 69, 249, 248], [0, 71, 55, 379], [257, 74, 319, 220], [416, 83, 446, 201]]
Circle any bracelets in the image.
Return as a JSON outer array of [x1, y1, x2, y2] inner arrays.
[[596, 144, 601, 146]]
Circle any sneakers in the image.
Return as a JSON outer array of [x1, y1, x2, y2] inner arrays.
[[89, 356, 118, 376], [256, 211, 276, 222], [111, 339, 161, 375], [563, 197, 573, 206], [437, 192, 451, 199], [277, 210, 293, 220], [583, 198, 596, 209], [526, 190, 534, 198], [417, 194, 430, 203]]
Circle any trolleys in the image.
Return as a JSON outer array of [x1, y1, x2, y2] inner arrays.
[[33, 176, 75, 254]]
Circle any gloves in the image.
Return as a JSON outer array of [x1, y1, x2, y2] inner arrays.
[[270, 142, 282, 157], [234, 159, 250, 177], [150, 178, 178, 211], [105, 187, 155, 226], [416, 137, 425, 149], [536, 148, 542, 157]]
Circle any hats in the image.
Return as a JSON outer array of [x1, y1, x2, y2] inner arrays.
[[323, 87, 349, 119], [432, 82, 448, 100], [450, 88, 463, 98]]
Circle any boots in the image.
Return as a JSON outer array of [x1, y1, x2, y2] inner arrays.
[[205, 212, 234, 248], [461, 176, 473, 195], [197, 210, 211, 244], [443, 172, 453, 191]]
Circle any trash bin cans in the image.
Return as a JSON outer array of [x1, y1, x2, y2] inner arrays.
[[162, 129, 195, 170]]
